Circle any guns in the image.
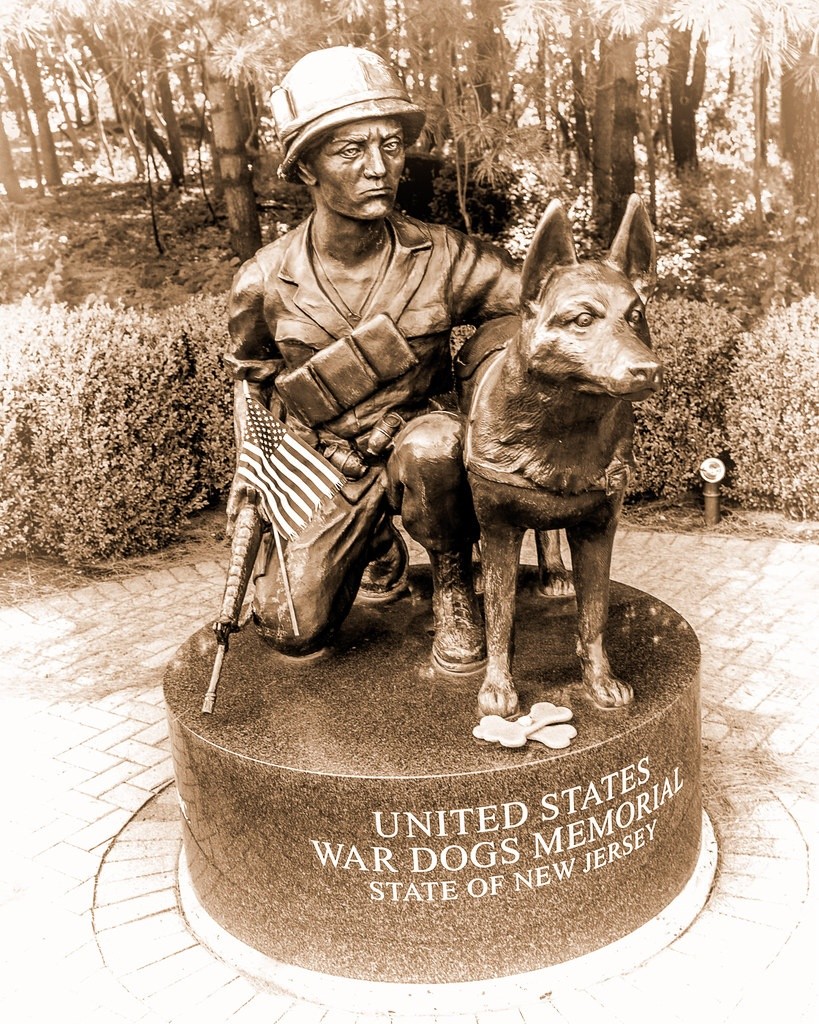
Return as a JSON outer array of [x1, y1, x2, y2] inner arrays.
[[201, 387, 293, 717]]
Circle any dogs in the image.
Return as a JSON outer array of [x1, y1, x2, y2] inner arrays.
[[453, 191, 665, 716]]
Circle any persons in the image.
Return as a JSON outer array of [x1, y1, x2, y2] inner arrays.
[[232, 44, 520, 675]]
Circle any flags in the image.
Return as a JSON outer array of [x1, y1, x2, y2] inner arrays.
[[236, 380, 346, 542]]
